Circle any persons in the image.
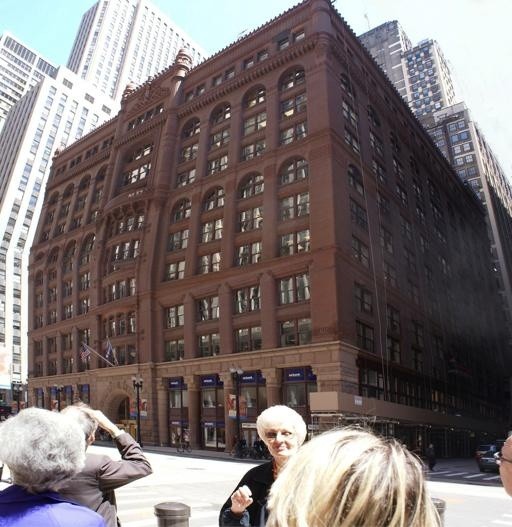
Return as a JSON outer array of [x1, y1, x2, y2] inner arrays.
[[183, 431, 192, 449], [2, 405, 110, 526], [57, 399, 153, 526], [264, 422, 442, 526], [218, 403, 308, 525], [426, 442, 437, 469], [492, 430, 511, 497], [1, 404, 14, 484]]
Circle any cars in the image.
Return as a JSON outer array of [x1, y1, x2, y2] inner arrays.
[[480, 450, 499, 471]]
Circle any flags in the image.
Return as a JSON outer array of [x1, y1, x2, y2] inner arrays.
[[81, 346, 90, 360], [105, 340, 111, 358]]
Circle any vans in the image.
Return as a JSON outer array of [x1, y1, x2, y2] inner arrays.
[[475, 445, 496, 460]]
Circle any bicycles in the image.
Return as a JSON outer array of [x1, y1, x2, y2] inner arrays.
[[176, 435, 192, 453]]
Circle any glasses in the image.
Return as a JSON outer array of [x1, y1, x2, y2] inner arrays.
[[494, 452, 512, 465]]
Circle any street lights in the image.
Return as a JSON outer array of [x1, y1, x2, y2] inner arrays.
[[132, 377, 144, 446], [14, 380, 23, 413], [56, 383, 65, 411], [230, 365, 244, 455]]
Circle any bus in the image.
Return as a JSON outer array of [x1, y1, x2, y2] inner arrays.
[[0, 399, 11, 422]]
